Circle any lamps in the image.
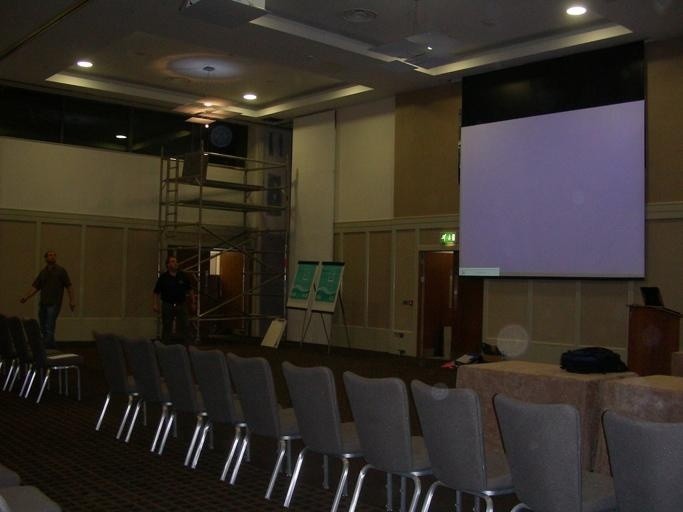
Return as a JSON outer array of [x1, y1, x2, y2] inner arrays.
[[164, 54, 244, 126]]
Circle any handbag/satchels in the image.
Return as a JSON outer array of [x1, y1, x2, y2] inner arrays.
[[560, 348, 629, 374]]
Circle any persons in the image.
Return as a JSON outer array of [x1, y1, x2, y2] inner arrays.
[[153, 255, 196, 342], [19, 250, 76, 348]]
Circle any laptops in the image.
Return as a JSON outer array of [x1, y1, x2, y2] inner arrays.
[[640, 286, 668, 308]]
